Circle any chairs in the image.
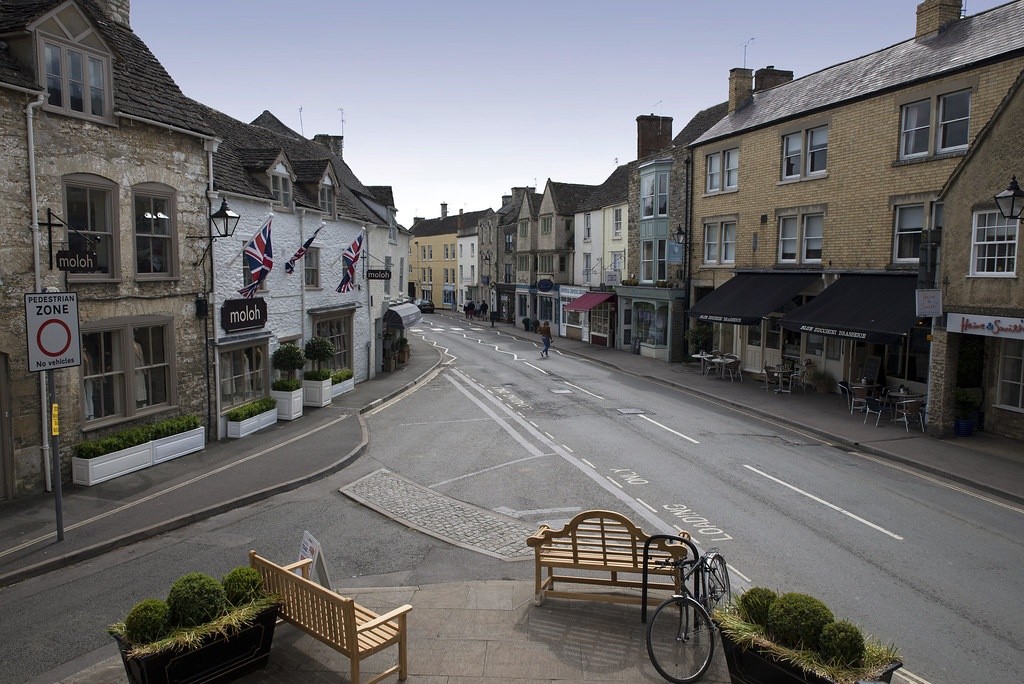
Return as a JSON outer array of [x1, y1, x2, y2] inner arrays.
[[764, 365, 808, 394], [837, 381, 925, 433], [704, 350, 743, 384]]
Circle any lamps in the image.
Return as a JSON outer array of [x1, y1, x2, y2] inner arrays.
[[993, 174, 1024, 224], [672, 224, 699, 245], [484, 255, 494, 265], [185, 196, 241, 266]]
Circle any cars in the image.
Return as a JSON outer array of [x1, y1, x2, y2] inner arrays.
[[415, 299, 435, 314]]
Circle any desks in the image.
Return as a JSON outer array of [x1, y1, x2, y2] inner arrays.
[[769, 368, 792, 394], [890, 392, 923, 426], [852, 383, 880, 413], [712, 359, 734, 381], [691, 354, 713, 375]]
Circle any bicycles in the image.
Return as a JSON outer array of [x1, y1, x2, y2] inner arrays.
[[643, 534, 731, 684]]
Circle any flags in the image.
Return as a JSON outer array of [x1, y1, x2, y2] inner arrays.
[[285, 226, 322, 274], [335, 233, 363, 293], [237, 218, 273, 299]]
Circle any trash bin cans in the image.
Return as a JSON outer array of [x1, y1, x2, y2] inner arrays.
[[490, 311, 497, 320]]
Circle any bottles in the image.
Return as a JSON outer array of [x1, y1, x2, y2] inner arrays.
[[780, 364, 782, 371], [782, 363, 785, 371], [719, 353, 723, 360]]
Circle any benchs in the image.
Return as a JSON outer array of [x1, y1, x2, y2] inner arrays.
[[526, 510, 692, 609], [244, 544, 414, 684]]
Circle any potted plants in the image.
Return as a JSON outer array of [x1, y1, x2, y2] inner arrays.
[[654, 280, 673, 288], [70, 412, 206, 487], [332, 368, 355, 397], [269, 343, 306, 420], [689, 326, 711, 364], [226, 398, 277, 439], [813, 371, 834, 393], [104, 565, 283, 684], [303, 337, 335, 408], [713, 582, 903, 684], [957, 381, 985, 436]]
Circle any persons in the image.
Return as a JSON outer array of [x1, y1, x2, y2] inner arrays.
[[134, 335, 147, 407], [222, 347, 264, 400], [463, 297, 488, 322], [533, 319, 540, 333], [540, 321, 553, 357], [82, 346, 96, 420]]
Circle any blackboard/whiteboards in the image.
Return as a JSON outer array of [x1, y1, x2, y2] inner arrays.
[[863, 356, 882, 382]]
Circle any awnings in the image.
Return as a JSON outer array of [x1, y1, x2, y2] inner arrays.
[[564, 292, 616, 313], [387, 302, 422, 330], [779, 275, 924, 346], [686, 273, 822, 326]]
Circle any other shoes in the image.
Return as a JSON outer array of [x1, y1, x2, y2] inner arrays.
[[540, 351, 544, 357], [545, 355, 550, 357]]
